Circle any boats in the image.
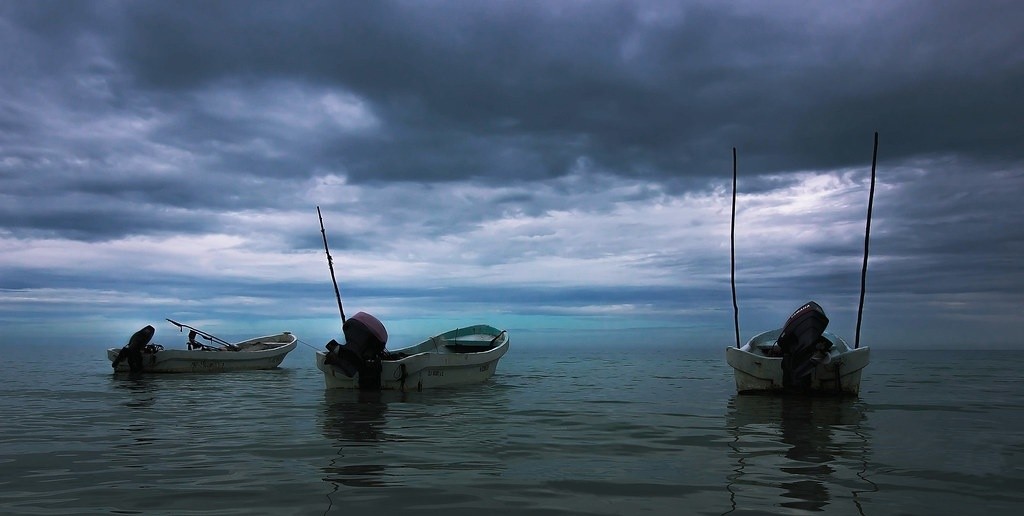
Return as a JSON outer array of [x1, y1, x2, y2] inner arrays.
[[313, 204, 511, 393], [725, 128, 882, 398], [107, 329, 297, 373]]
